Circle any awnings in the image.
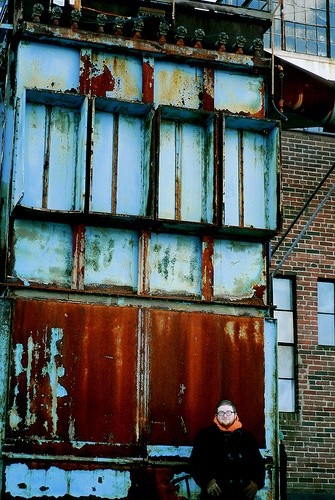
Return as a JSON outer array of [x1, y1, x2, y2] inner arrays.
[[266, 50, 335, 86]]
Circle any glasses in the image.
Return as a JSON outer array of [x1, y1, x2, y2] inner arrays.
[[216, 411, 234, 416]]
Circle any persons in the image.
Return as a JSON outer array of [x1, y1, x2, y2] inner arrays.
[[189, 399, 266, 500]]
